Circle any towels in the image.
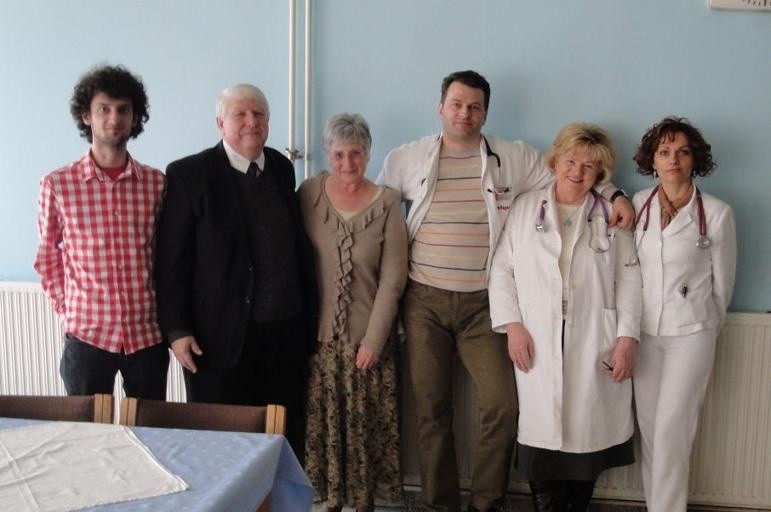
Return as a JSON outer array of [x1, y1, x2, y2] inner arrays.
[[0, 422, 190, 512]]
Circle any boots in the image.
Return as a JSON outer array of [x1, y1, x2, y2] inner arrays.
[[526, 479, 594, 512]]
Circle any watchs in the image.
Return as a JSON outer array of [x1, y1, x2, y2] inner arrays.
[[608, 189, 630, 204]]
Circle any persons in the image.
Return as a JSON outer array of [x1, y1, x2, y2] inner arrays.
[[629, 114, 738, 511], [377, 69, 636, 512], [152, 84, 305, 466], [486, 122, 646, 510], [293, 111, 410, 512], [32, 65, 169, 399]]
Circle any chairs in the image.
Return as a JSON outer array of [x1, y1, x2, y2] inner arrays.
[[0, 394, 114, 423], [118, 396, 286, 512]]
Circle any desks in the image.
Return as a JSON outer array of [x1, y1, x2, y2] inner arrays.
[[0, 418, 313, 512]]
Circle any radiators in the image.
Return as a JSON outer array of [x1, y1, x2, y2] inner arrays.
[[395, 313, 771, 512], [0, 281, 187, 423]]
